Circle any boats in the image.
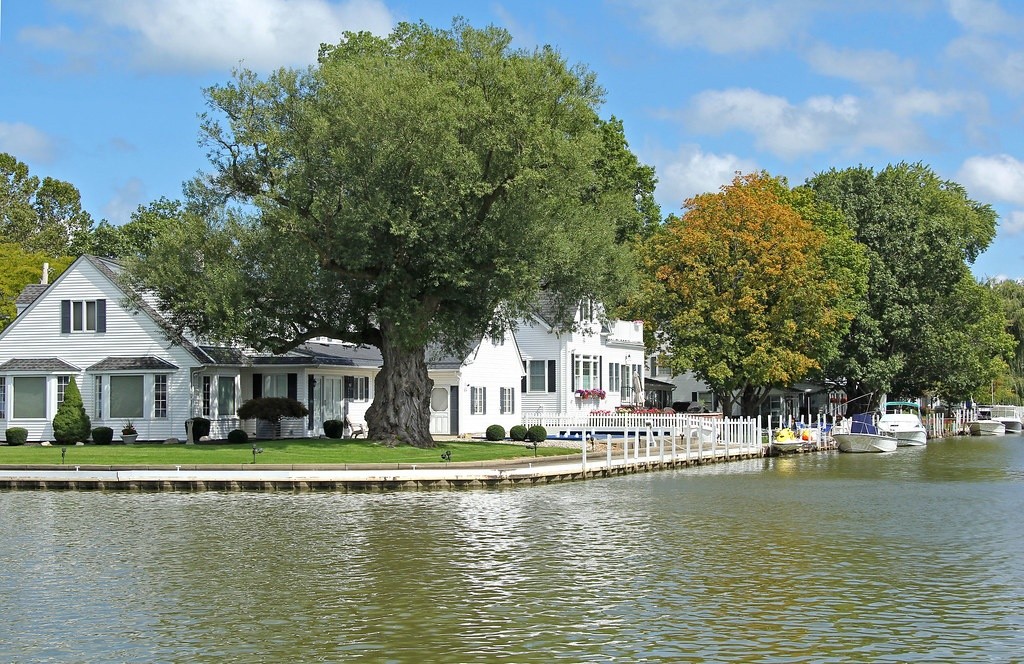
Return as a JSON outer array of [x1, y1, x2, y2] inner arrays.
[[967, 419, 1006, 436], [876, 401, 927, 445], [771, 428, 808, 453], [991, 402, 1023, 433], [831, 411, 898, 454]]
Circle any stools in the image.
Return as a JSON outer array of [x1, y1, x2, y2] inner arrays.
[[345, 418, 368, 439]]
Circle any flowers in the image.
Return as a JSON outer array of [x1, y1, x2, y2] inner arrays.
[[121, 418, 138, 435], [576, 389, 606, 399]]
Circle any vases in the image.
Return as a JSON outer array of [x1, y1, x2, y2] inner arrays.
[[120, 434, 139, 445]]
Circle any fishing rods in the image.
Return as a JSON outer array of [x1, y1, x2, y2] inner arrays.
[[797, 389, 879, 440]]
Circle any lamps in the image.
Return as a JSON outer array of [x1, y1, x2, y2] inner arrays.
[[312, 379, 316, 387], [587, 437, 594, 452], [252, 444, 263, 464], [441, 451, 451, 462], [526, 441, 537, 458]]
